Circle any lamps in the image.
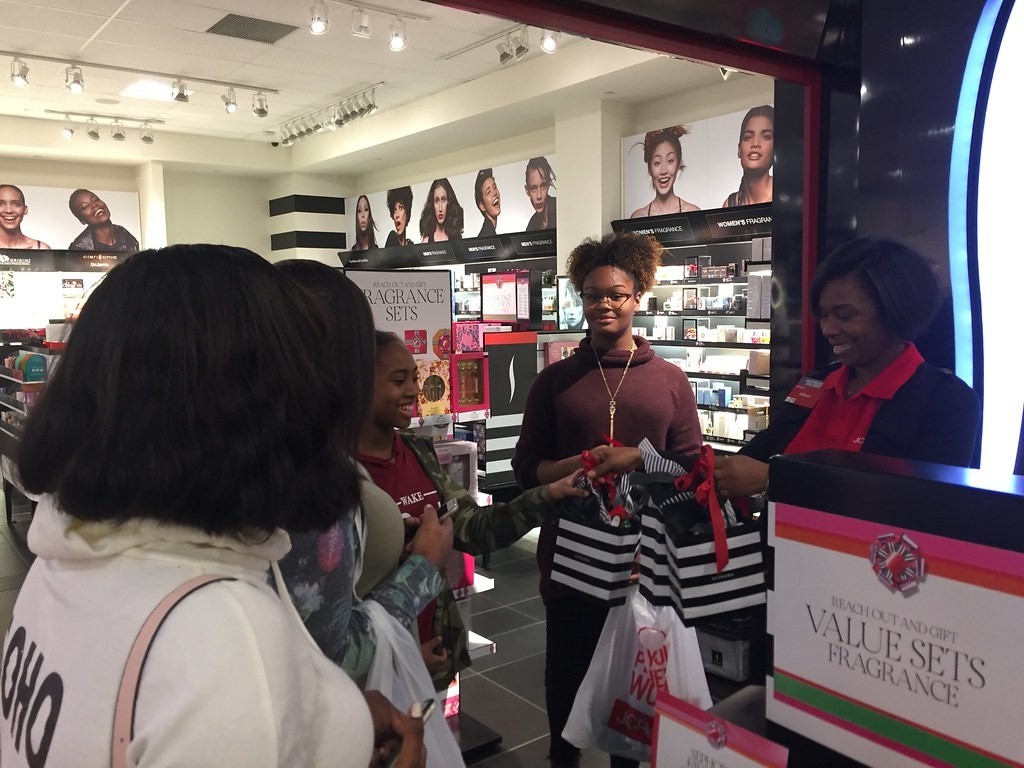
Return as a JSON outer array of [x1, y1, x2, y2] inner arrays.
[[171, 79, 190, 103], [110, 119, 126, 140], [62, 115, 76, 139], [138, 122, 153, 144], [220, 86, 239, 114], [512, 25, 530, 62], [251, 90, 270, 119], [495, 31, 513, 66], [349, 4, 373, 41], [309, 1, 331, 35], [265, 82, 382, 152], [9, 54, 30, 89], [86, 116, 101, 140], [387, 15, 409, 53], [64, 64, 85, 92], [538, 28, 559, 55]]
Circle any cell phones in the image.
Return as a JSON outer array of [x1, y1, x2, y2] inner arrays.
[[404, 497, 459, 545], [369, 698, 436, 768]]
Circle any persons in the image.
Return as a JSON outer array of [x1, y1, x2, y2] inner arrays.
[[358, 330, 587, 692], [0, 242, 374, 767], [384, 185, 415, 248], [474, 168, 500, 238], [0, 185, 52, 249], [631, 124, 699, 218], [65, 189, 139, 251], [510, 233, 705, 768], [524, 156, 555, 232], [419, 178, 464, 244], [586, 234, 980, 495], [722, 106, 772, 208], [352, 195, 379, 251], [271, 259, 453, 690]]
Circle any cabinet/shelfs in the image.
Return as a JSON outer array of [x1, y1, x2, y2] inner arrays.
[[611, 199, 774, 452], [1, 317, 75, 525], [339, 223, 558, 717]]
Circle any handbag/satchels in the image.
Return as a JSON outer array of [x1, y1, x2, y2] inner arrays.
[[552, 510, 637, 607], [638, 503, 670, 608], [560, 589, 713, 763], [666, 499, 767, 629], [356, 596, 468, 767]]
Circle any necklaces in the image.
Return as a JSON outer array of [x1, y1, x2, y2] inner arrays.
[[589, 340, 634, 449]]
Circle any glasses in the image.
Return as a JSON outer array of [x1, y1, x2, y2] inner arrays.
[[579, 289, 637, 308]]
